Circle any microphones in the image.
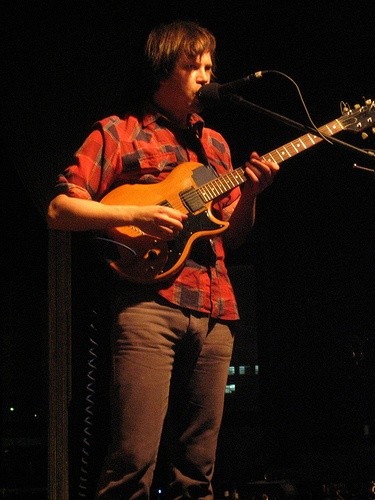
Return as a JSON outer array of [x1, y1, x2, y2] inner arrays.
[[197, 70, 268, 112]]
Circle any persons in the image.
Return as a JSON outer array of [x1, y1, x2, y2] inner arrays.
[[46, 18, 281, 500]]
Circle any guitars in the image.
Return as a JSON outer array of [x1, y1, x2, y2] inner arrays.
[[104, 99, 375, 283]]
